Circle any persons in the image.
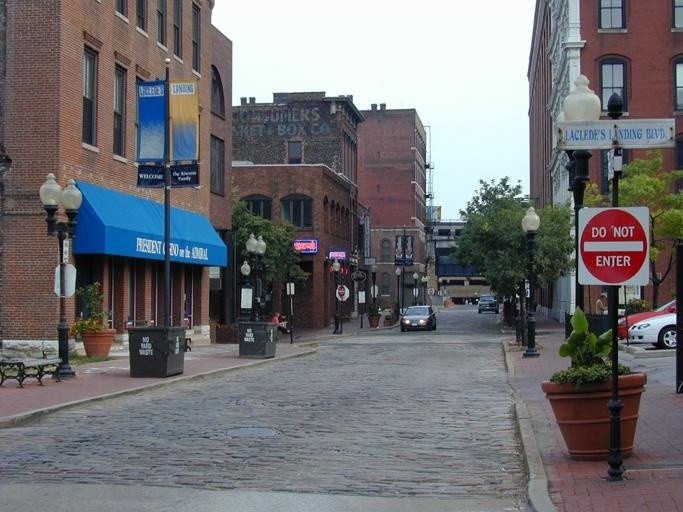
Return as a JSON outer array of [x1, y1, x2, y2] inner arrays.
[[596, 293, 608, 314], [271, 312, 291, 334]]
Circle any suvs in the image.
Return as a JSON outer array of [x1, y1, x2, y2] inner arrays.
[[474, 294, 499, 315]]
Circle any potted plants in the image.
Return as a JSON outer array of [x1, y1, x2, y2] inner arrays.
[[367, 300, 384, 328], [68, 279, 118, 359], [384, 312, 395, 327], [538, 305, 649, 463]]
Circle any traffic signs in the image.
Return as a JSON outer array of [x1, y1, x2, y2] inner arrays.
[[556, 115, 683, 155]]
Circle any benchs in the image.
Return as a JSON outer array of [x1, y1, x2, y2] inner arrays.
[[1, 340, 65, 387]]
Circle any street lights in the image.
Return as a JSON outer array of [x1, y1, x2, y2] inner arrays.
[[240, 259, 253, 291], [245, 233, 266, 321], [370, 263, 379, 306], [411, 271, 418, 305], [552, 71, 602, 326], [523, 206, 542, 358], [38, 168, 86, 379], [394, 265, 401, 313], [436, 276, 450, 298], [331, 254, 342, 335]]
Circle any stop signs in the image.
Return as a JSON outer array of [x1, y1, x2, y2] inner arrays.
[[427, 287, 434, 295], [575, 206, 650, 286], [337, 286, 345, 298]]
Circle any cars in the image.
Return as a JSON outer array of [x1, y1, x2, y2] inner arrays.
[[616, 298, 676, 340], [628, 313, 676, 350], [398, 304, 436, 333]]
